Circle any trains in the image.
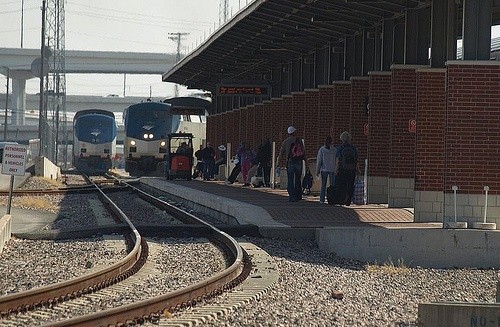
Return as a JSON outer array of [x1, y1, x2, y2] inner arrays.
[[123, 97, 175, 176], [71, 108, 117, 174]]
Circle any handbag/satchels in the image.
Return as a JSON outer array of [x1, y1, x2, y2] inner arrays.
[[290, 136, 305, 158]]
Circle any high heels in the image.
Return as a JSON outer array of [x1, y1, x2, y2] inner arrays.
[[320, 200, 324, 204]]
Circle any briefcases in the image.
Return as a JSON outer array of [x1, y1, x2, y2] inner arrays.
[[326, 185, 354, 206]]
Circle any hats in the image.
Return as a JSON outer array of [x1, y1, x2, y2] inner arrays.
[[287, 126, 296, 133], [218, 144, 227, 150]]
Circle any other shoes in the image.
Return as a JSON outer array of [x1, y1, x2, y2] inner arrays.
[[242, 182, 251, 186], [289, 196, 302, 201], [204, 176, 210, 180]]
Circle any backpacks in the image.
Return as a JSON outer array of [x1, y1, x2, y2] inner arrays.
[[302, 166, 313, 188]]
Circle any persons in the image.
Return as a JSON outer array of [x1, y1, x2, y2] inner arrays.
[[176, 134, 273, 188], [334, 131, 362, 206], [316, 134, 339, 206], [276, 126, 313, 202]]
